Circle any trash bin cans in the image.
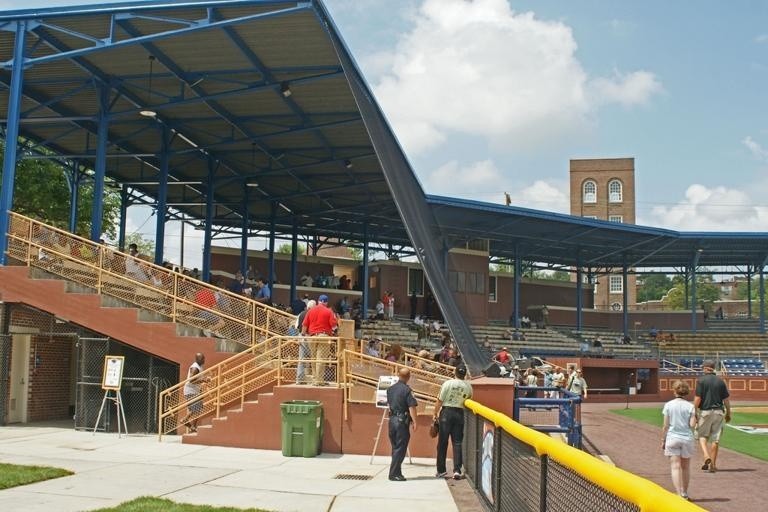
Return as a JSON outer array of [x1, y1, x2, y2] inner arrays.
[[280, 400, 324, 457]]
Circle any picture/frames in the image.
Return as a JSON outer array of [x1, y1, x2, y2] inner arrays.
[[102, 356, 124, 390]]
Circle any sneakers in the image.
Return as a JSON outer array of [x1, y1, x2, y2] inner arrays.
[[180, 418, 191, 428], [701, 457, 710, 470], [435, 472, 459, 479], [187, 428, 196, 434]]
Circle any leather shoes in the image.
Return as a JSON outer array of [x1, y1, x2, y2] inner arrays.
[[390, 476, 406, 481]]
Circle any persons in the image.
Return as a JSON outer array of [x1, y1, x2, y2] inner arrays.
[[480, 329, 587, 412], [616, 335, 623, 344], [624, 372, 634, 394], [35, 222, 366, 385], [376, 288, 395, 320], [410, 288, 445, 340], [693, 358, 731, 472], [180, 353, 212, 433], [649, 325, 677, 342], [367, 338, 462, 378], [510, 313, 545, 329], [715, 307, 723, 320], [594, 333, 601, 347], [432, 363, 473, 479], [386, 367, 418, 482], [623, 334, 632, 345], [662, 380, 696, 499]]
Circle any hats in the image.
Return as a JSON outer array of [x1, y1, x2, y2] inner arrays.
[[699, 360, 714, 368], [455, 364, 465, 374], [418, 350, 430, 358], [318, 294, 328, 302]]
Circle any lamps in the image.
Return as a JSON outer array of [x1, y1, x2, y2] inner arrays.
[[246, 143, 257, 186], [139, 56, 157, 116]]
